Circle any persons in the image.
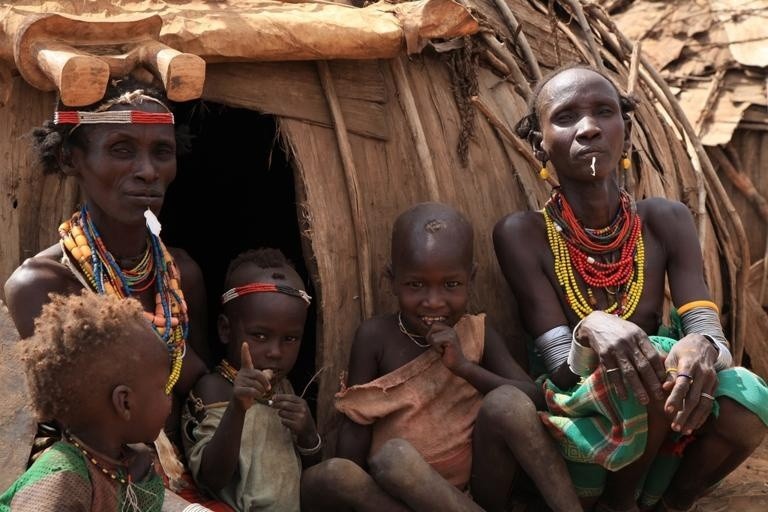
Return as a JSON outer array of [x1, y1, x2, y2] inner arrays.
[[2, 290, 174, 512], [2, 75, 208, 472], [165, 246, 206, 450], [339, 202, 582, 512], [182, 247, 401, 512], [491, 65, 766, 512]]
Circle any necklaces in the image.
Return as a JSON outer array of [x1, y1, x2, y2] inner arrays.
[[55, 212, 188, 398], [538, 185, 643, 321], [59, 426, 156, 512]]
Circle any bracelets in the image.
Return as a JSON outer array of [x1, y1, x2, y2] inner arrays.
[[677, 299, 733, 371], [532, 325, 598, 379], [294, 434, 324, 456]]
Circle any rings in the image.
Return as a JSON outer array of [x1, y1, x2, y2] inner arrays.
[[679, 374, 693, 383], [665, 368, 676, 374], [701, 391, 715, 399], [607, 369, 618, 374]]
